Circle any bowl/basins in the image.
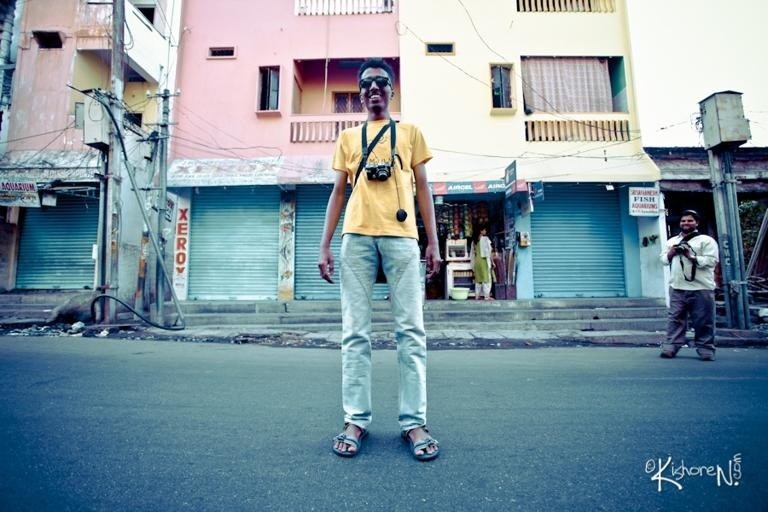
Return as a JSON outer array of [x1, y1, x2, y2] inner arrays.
[[450, 287, 470, 301]]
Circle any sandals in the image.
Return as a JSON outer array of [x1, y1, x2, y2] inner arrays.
[[331, 422, 368, 458], [401, 425, 440, 461]]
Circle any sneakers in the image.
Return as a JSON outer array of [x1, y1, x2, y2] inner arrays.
[[660, 350, 676, 358], [701, 350, 716, 361]]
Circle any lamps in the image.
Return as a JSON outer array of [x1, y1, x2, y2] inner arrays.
[[435, 196, 443, 204], [606, 184, 614, 190]]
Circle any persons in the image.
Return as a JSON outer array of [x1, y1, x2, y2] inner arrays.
[[316, 54, 444, 463], [468, 223, 495, 302], [655, 208, 720, 362]]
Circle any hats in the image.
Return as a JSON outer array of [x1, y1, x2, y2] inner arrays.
[[678, 209, 700, 221]]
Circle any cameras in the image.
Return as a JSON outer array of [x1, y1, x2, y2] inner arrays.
[[366, 162, 393, 183], [675, 243, 691, 256]]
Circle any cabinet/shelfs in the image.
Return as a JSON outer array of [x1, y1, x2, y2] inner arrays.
[[444, 258, 475, 300]]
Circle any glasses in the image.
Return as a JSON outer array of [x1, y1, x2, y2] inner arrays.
[[359, 75, 392, 92]]
